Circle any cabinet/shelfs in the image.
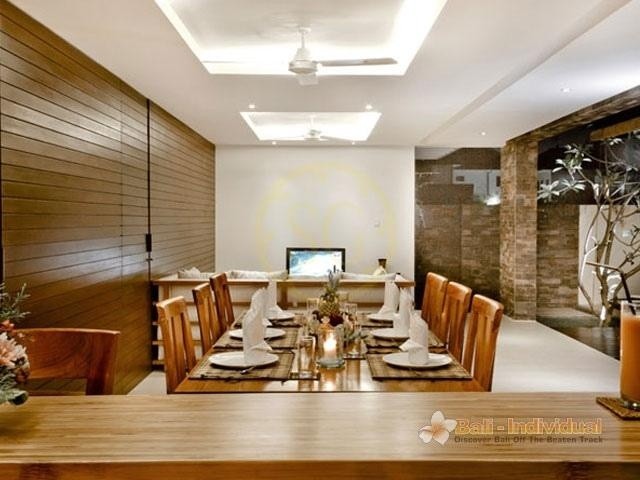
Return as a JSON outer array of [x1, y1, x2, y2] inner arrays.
[[152, 272, 415, 371]]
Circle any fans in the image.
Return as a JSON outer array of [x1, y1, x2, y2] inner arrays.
[[199, 26, 397, 86], [280, 116, 350, 141]]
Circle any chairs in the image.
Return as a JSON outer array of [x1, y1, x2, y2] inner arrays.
[[421, 272, 507, 391], [154, 274, 236, 393], [7, 327, 119, 396]]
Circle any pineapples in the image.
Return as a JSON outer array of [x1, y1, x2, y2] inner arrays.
[[319, 265, 342, 316]]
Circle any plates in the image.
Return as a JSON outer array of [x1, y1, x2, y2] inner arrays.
[[366, 312, 398, 322], [229, 328, 286, 339], [208, 350, 280, 368], [267, 312, 295, 320], [370, 327, 410, 339], [382, 351, 452, 368]]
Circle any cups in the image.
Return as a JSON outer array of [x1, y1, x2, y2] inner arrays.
[[620, 299, 640, 411], [298, 297, 361, 377]]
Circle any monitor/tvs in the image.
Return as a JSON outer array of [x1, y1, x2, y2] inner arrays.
[[286, 247, 346, 275]]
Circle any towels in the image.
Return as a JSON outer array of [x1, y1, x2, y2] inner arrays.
[[242, 274, 430, 365]]
